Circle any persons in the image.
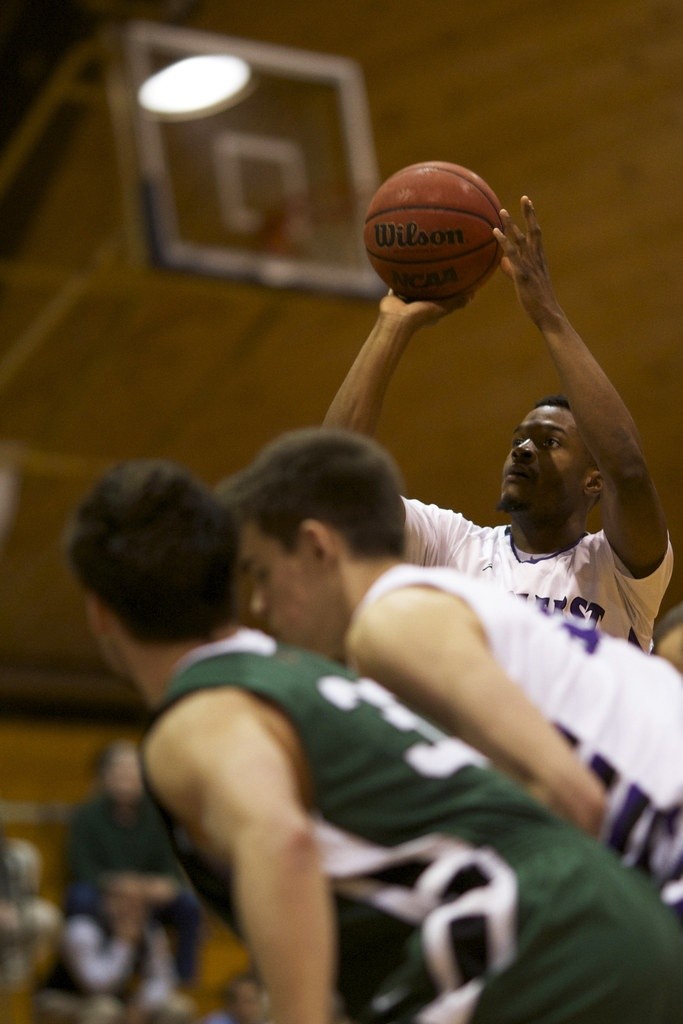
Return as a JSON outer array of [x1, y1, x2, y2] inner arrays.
[[0, 427, 682, 1023], [326, 197, 673, 659]]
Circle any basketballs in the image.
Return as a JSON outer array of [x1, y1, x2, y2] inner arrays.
[[361, 159, 508, 303]]
